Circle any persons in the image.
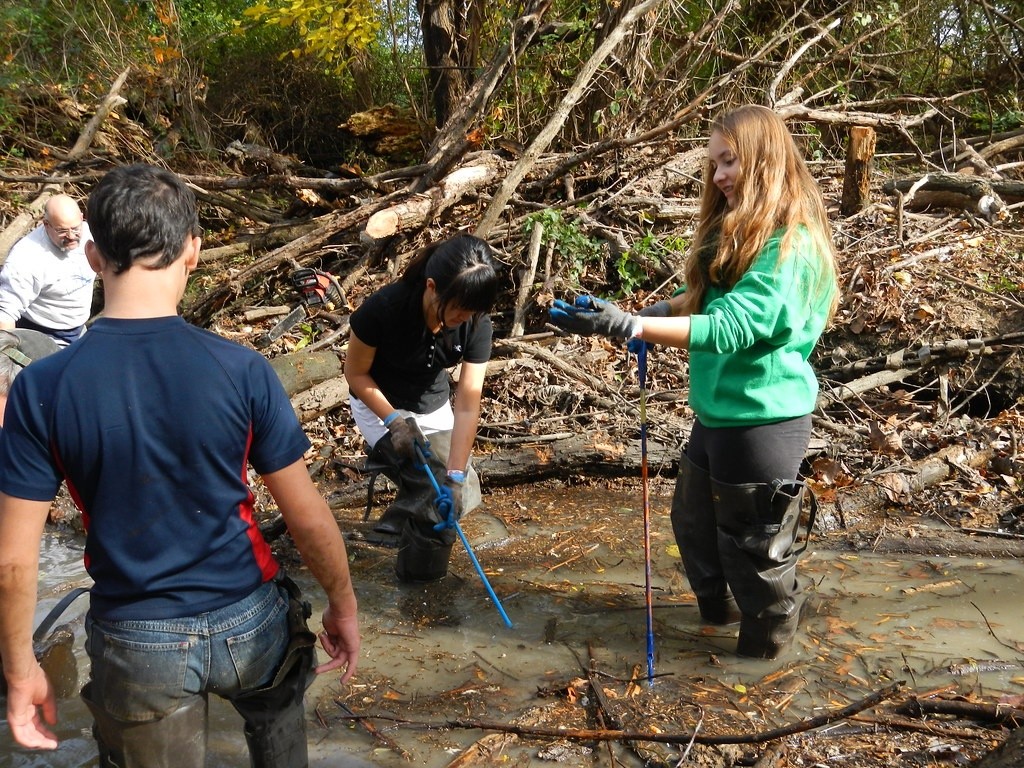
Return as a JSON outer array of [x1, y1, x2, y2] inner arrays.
[[0, 164, 362, 768], [0, 329, 64, 427], [344, 235, 501, 583], [0, 195, 98, 350], [547, 104, 845, 660]]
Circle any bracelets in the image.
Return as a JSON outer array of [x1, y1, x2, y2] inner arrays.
[[634, 314, 643, 339], [446, 470, 463, 477], [383, 413, 399, 426]]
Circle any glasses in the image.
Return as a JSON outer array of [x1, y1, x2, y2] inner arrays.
[[46, 218, 82, 237]]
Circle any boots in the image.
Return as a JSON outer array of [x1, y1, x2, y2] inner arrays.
[[707, 473, 816, 660], [671, 444, 731, 624], [366, 419, 461, 586], [366, 427, 481, 548]]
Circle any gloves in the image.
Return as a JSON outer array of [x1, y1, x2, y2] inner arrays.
[[384, 412, 432, 473], [547, 294, 640, 343], [625, 299, 674, 354], [430, 473, 464, 531]]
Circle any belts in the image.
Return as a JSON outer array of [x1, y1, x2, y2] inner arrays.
[[33, 587, 90, 641]]
